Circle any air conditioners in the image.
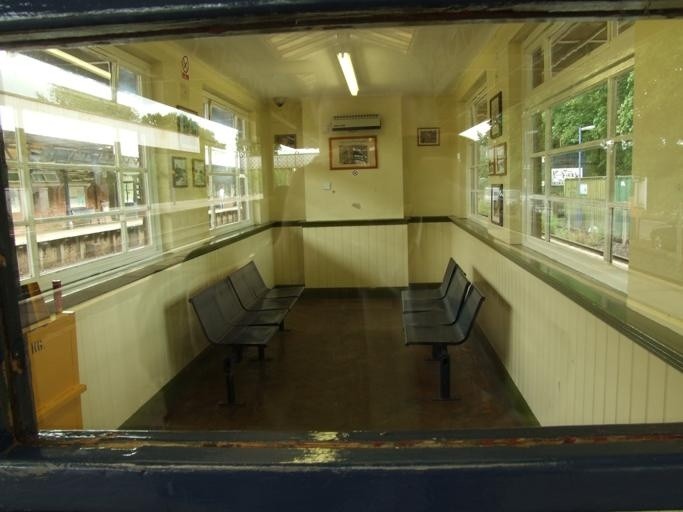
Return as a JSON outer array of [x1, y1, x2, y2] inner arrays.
[[332, 114, 381, 129]]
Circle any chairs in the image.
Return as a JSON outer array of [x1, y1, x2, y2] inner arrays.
[[401, 257, 486, 402], [187, 260, 305, 406]]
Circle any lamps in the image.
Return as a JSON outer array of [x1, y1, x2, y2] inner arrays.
[[337, 47, 359, 96]]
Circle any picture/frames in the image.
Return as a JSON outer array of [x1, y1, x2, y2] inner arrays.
[[487, 91, 507, 226], [417, 127, 440, 145], [274, 134, 296, 149], [329, 135, 380, 170], [172, 105, 205, 187]]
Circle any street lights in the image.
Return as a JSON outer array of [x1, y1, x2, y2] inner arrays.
[[578, 123, 597, 177]]
